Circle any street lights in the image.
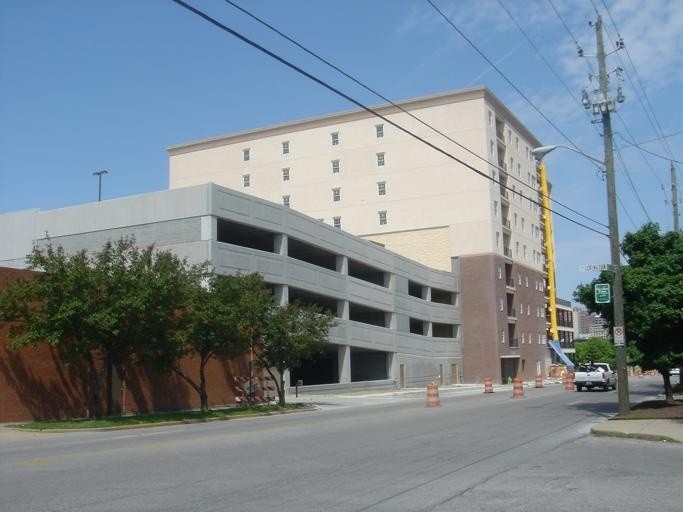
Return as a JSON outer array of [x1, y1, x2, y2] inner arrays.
[[529, 142, 632, 412], [90, 168, 109, 202]]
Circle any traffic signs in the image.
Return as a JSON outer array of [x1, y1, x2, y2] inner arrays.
[[605, 264, 617, 273], [593, 283, 611, 304], [576, 263, 619, 273]]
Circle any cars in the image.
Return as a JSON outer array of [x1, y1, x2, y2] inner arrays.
[[667, 368, 680, 376]]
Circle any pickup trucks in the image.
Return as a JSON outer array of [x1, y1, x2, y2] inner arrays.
[[572, 360, 617, 393]]
